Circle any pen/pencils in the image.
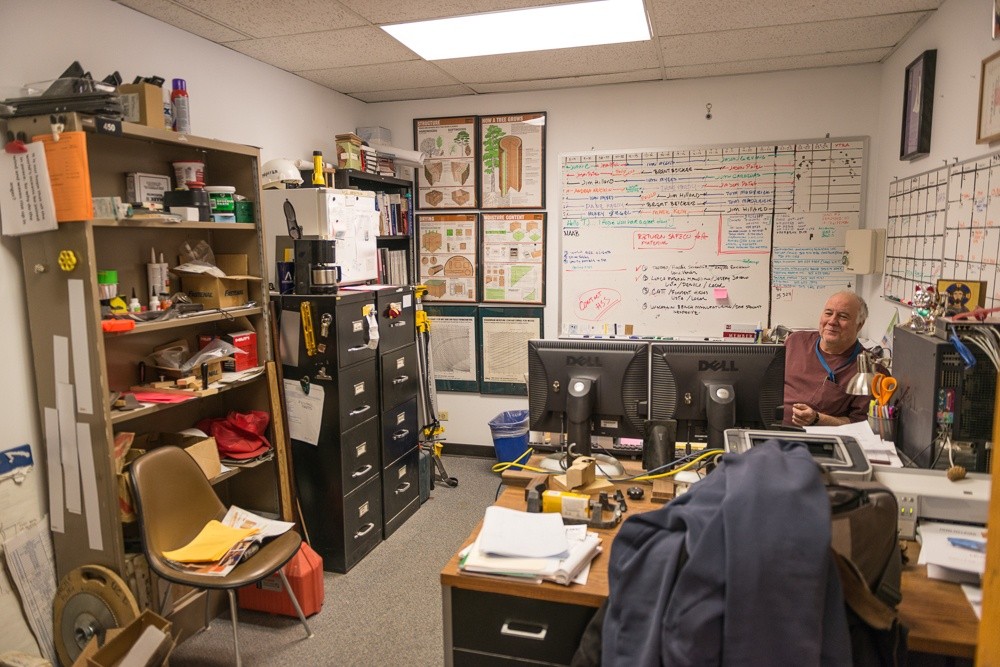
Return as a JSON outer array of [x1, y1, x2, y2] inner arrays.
[[823, 376, 827, 385]]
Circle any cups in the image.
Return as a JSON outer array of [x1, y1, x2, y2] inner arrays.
[[277, 262, 295, 295]]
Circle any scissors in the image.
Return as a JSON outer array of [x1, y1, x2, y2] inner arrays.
[[872, 373, 897, 437]]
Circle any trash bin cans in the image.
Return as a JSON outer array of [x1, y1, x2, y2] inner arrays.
[[488, 410, 531, 471]]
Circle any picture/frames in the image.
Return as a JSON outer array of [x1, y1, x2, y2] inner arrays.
[[900, 48, 937, 161], [976, 49, 1000, 144]]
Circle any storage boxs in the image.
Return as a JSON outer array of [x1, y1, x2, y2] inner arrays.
[[159, 361, 223, 388], [125, 172, 170, 211], [337, 143, 361, 171], [87, 608, 172, 667], [71, 627, 184, 667], [172, 253, 262, 310], [120, 82, 164, 128], [356, 126, 391, 146], [197, 316, 258, 372], [128, 433, 220, 482]]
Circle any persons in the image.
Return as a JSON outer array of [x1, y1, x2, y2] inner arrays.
[[782, 290, 877, 426]]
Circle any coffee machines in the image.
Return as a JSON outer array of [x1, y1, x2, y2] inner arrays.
[[293, 239, 341, 295]]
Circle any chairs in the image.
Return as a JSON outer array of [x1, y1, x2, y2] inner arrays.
[[134, 445, 314, 667]]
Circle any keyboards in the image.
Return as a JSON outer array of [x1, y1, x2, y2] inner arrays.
[[675, 449, 706, 461], [527, 441, 644, 460]]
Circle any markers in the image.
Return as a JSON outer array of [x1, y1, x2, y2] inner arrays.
[[885, 405, 892, 435], [879, 406, 883, 437], [900, 299, 911, 305], [569, 335, 589, 338], [867, 399, 899, 439], [589, 336, 601, 338], [615, 336, 679, 340], [889, 296, 899, 301], [704, 338, 725, 341], [601, 336, 615, 338]]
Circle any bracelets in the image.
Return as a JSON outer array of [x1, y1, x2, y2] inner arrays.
[[809, 412, 819, 426]]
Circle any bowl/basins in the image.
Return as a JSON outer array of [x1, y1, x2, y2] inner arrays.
[[356, 126, 392, 146], [24, 77, 125, 120]]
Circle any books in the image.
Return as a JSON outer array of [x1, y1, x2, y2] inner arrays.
[[374, 190, 413, 286]]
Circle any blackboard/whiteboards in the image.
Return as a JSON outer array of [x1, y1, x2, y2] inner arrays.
[[880, 150, 1000, 328], [557, 136, 869, 346]]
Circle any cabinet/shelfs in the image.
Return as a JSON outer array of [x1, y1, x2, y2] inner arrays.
[[8, 108, 292, 646], [340, 284, 420, 539], [443, 587, 599, 667], [269, 284, 383, 574], [298, 169, 415, 287]]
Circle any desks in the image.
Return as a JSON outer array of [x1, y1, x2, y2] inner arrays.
[[440, 451, 978, 657]]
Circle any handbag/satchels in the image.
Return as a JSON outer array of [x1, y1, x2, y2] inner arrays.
[[826, 479, 901, 614]]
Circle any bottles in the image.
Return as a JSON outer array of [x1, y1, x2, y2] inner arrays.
[[158, 293, 173, 311], [129, 287, 140, 312], [148, 247, 170, 298], [171, 78, 190, 134], [162, 87, 178, 129], [754, 321, 763, 344], [149, 285, 161, 311]]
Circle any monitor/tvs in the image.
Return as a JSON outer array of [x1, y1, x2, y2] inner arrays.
[[527, 338, 786, 475]]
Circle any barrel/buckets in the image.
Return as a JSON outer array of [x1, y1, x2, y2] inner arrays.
[[204, 185, 236, 212], [172, 159, 206, 190]]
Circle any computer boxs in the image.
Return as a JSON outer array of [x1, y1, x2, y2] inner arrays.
[[892, 322, 998, 472]]
